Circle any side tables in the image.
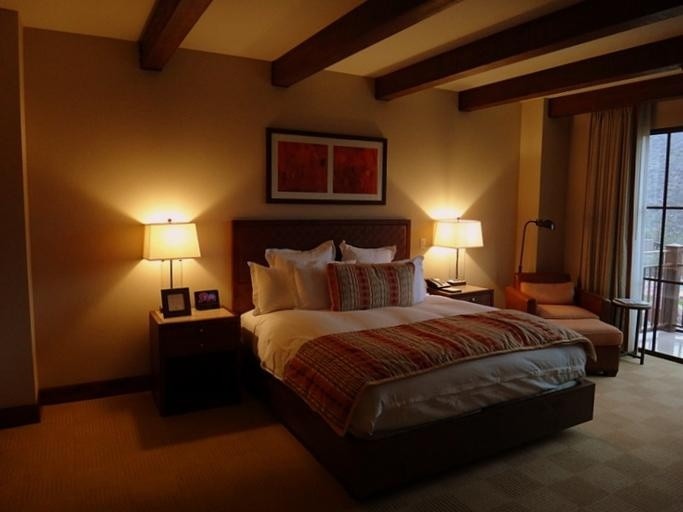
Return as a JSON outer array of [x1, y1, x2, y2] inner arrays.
[[612, 299, 651, 365]]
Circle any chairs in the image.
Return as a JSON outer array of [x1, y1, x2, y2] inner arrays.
[[504, 272, 611, 324]]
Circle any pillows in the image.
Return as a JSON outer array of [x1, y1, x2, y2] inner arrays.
[[247, 240, 427, 316], [520, 281, 577, 305]]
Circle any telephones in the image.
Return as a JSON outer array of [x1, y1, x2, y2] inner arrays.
[[424, 277, 451, 288]]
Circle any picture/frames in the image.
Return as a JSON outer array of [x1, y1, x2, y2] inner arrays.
[[161, 287, 192, 319], [265, 127, 388, 205], [194, 289, 220, 310]]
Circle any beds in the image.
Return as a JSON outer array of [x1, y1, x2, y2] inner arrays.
[[231, 218, 596, 505]]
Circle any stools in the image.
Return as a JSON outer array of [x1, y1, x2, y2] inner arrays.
[[543, 319, 624, 377]]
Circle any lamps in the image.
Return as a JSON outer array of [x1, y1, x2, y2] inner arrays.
[[142, 219, 202, 313], [519, 218, 555, 272]]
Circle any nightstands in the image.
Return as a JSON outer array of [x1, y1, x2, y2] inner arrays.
[[426, 285, 494, 309], [149, 306, 240, 418]]
